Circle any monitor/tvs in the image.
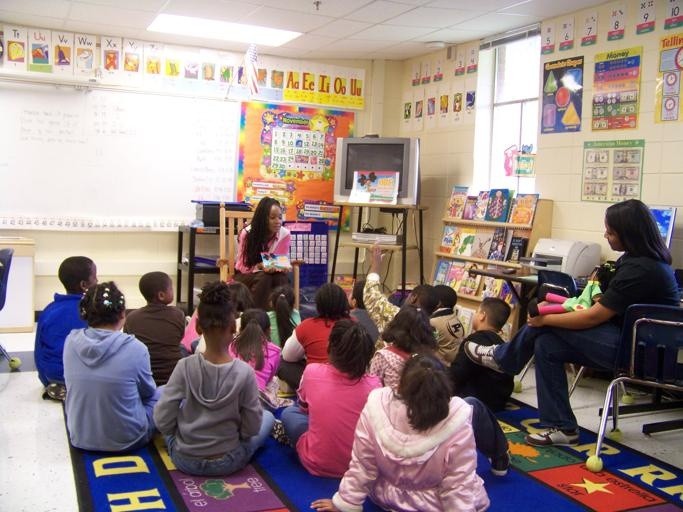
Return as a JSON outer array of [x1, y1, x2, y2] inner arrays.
[[333, 136, 421, 206], [648, 207, 677, 248]]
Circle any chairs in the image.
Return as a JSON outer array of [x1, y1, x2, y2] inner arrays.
[[0, 248, 22, 371], [586, 304, 683, 473], [513, 269, 588, 392], [219, 200, 304, 313]]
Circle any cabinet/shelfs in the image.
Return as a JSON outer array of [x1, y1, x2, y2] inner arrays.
[[426, 199, 553, 327], [331, 202, 430, 298]]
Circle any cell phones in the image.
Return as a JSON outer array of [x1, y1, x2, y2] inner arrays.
[[502, 268, 517, 274]]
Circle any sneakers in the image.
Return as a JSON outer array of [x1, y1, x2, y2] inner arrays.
[[525, 428, 580, 446], [464, 341, 504, 373], [488, 448, 510, 476]]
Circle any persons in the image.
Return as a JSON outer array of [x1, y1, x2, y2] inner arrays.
[[120, 273, 303, 408], [153, 281, 276, 476], [233, 196, 291, 308], [272, 321, 383, 477], [311, 353, 511, 512], [34, 256, 98, 401], [63, 282, 168, 456], [463, 199, 683, 448], [277, 246, 514, 411], [530, 259, 615, 318]]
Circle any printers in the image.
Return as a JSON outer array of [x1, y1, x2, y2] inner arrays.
[[518, 238, 602, 280]]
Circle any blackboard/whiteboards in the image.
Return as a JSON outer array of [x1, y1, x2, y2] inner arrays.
[[2, 75, 241, 231]]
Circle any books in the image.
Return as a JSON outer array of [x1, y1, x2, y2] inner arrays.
[[432, 185, 539, 342]]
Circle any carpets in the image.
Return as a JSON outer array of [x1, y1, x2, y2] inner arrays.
[[61, 398, 683, 512]]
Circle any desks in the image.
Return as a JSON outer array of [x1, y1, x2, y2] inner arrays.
[[0, 236, 36, 334], [470, 269, 584, 330], [176, 225, 237, 317]]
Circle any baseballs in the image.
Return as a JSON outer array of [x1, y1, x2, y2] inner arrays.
[[10, 357, 21, 370]]
